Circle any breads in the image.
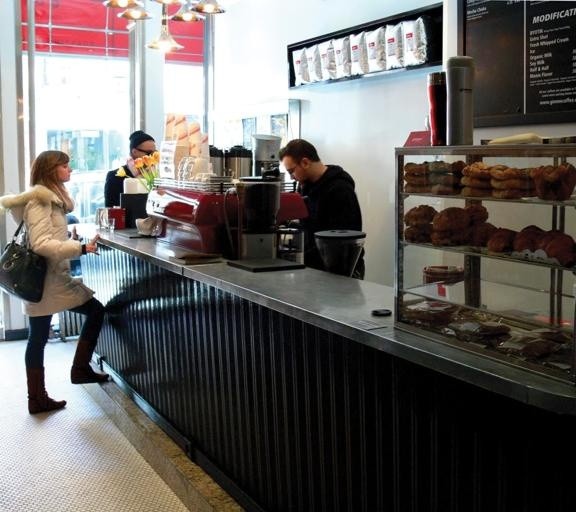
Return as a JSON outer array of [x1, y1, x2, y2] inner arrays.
[[404, 202, 576, 267]]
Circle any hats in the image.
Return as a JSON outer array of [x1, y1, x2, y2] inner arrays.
[[129, 130, 155, 152]]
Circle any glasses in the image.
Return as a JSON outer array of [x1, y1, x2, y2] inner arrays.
[[134, 147, 157, 156]]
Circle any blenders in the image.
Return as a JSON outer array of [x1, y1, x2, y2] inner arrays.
[[222, 176, 283, 261]]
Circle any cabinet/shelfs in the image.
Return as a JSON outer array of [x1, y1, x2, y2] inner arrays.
[[394, 142, 576, 386]]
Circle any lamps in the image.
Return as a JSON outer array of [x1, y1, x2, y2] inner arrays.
[[102, 0, 226, 50]]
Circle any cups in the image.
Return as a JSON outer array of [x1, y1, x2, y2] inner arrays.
[[425, 54, 477, 146], [104, 218, 115, 230]]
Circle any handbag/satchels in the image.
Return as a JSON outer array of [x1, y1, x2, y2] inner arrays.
[[0, 243, 46, 302]]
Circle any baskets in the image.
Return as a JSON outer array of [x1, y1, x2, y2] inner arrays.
[[153, 176, 296, 196]]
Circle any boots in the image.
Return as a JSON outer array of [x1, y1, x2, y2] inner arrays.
[[71, 336, 109, 384], [26, 367, 66, 414]]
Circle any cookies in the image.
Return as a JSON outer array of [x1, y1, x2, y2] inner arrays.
[[404, 159, 576, 199]]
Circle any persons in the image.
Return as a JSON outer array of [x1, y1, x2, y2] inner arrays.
[[277, 139, 365, 280], [0, 149, 110, 415], [104, 130, 158, 229]]
